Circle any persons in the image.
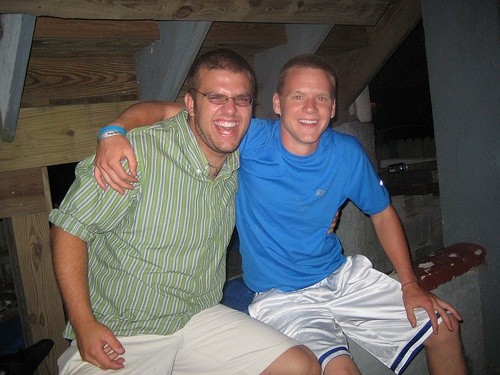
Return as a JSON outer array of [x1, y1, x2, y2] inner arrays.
[[48, 48, 338, 375], [94, 53, 468, 375]]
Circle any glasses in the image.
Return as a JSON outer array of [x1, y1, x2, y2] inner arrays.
[[189, 87, 256, 107]]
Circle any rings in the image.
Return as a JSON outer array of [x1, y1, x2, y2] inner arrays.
[[96, 364, 101, 367]]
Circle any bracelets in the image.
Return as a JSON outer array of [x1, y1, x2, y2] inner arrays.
[[97, 126, 126, 141], [401, 280, 419, 290]]
